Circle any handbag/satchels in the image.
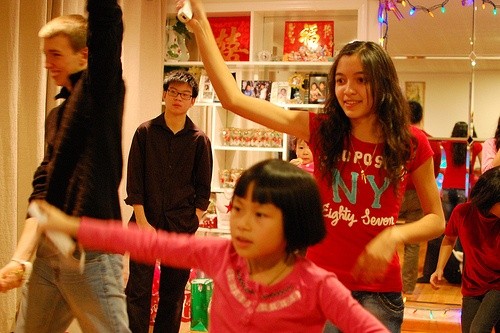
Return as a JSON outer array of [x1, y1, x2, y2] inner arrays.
[[190, 278, 214, 332]]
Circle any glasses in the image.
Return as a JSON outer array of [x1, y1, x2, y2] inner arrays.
[[167, 88, 193, 101]]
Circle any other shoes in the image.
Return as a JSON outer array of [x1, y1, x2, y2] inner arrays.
[[416, 276, 432, 284]]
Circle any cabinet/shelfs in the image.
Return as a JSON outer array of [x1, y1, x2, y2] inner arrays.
[[160, 0, 368, 234]]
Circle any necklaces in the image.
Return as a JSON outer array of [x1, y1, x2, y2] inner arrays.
[[347, 132, 382, 183], [246, 251, 293, 286]]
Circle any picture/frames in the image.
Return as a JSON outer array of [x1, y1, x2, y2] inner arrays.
[[271, 82, 291, 105], [241, 80, 272, 103], [308, 73, 329, 104]]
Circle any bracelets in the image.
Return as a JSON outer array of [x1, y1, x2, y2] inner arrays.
[[10, 258, 27, 270]]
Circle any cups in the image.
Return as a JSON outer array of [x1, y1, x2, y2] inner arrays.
[[219, 168, 244, 188], [222, 127, 282, 147]]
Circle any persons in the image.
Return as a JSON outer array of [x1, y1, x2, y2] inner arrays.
[[29, 160, 391, 333], [0, 0, 133, 333], [290, 137, 315, 176], [124, 70, 214, 333], [244, 81, 327, 105], [175, 0, 446, 333], [398, 101, 500, 333]]
